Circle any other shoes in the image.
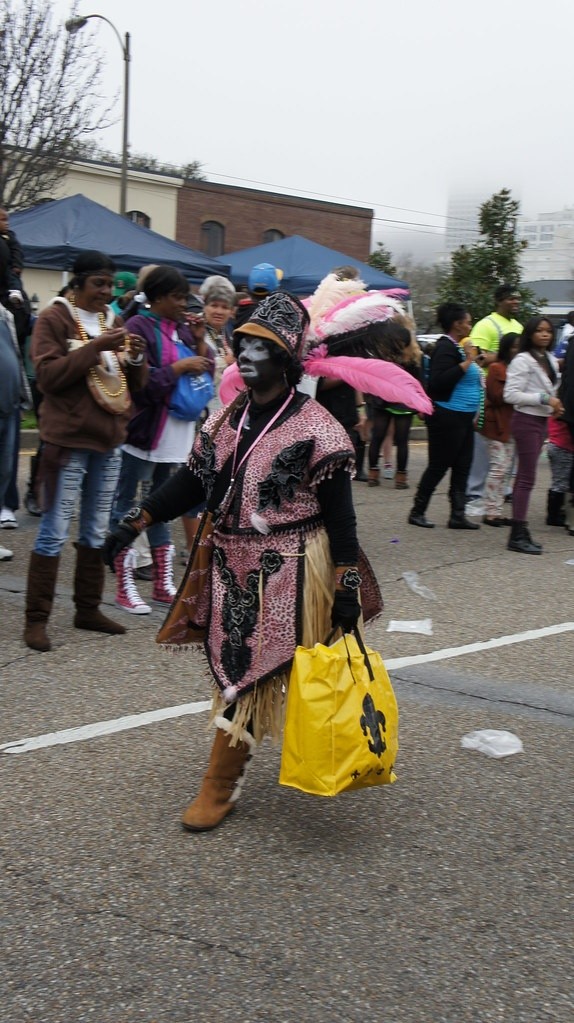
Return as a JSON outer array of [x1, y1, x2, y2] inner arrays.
[[483, 516, 514, 528], [448, 516, 481, 530], [408, 514, 434, 528], [505, 492, 513, 502], [385, 468, 393, 479], [0, 546, 14, 562], [352, 472, 368, 482], [466, 494, 483, 504], [24, 495, 42, 517]]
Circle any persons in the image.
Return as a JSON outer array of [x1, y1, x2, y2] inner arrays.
[[105, 263, 283, 614], [296, 266, 435, 490], [102, 291, 386, 833], [407, 285, 574, 555], [0, 207, 147, 652]]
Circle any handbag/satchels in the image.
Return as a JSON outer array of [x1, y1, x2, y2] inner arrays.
[[167, 341, 218, 422], [278, 625, 398, 796]]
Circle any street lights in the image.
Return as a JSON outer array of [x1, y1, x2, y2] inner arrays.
[[65, 14, 130, 217]]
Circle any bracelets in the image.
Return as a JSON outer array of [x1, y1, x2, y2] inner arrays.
[[542, 393, 550, 405]]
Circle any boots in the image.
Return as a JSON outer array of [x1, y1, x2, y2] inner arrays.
[[23, 551, 61, 651], [149, 544, 179, 602], [394, 469, 409, 489], [71, 540, 126, 634], [367, 467, 380, 486], [181, 713, 257, 833], [507, 520, 542, 555], [545, 487, 568, 526]]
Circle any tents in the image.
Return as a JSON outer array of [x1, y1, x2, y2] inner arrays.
[[186, 235, 410, 295], [8, 193, 232, 289]]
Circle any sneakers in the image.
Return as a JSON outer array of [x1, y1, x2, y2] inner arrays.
[[0, 502, 19, 528]]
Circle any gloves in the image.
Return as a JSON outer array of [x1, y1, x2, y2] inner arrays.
[[331, 590, 362, 633], [102, 523, 137, 565]]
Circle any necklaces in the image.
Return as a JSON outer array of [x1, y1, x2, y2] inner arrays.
[[203, 313, 233, 366], [210, 386, 295, 524], [70, 295, 126, 397], [444, 335, 486, 430]]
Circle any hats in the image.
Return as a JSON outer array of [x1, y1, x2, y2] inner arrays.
[[232, 290, 309, 384]]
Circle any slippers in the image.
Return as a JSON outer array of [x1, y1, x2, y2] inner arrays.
[[112, 545, 153, 613]]
